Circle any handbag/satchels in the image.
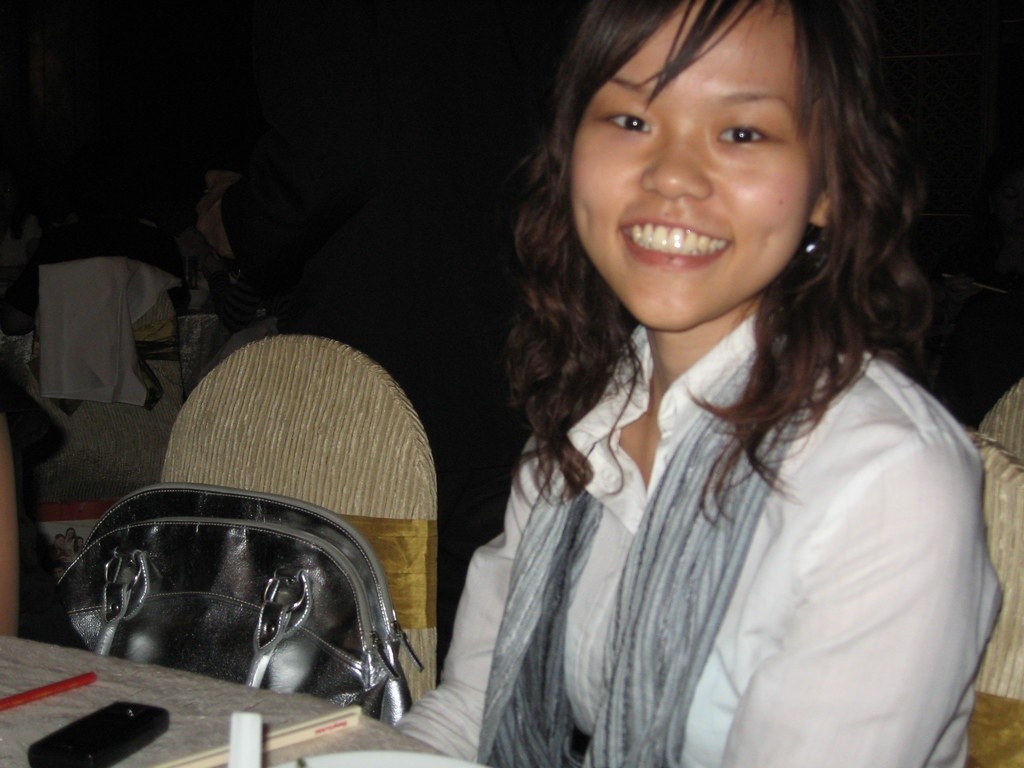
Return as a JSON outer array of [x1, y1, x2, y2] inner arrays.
[[57, 481, 423, 727]]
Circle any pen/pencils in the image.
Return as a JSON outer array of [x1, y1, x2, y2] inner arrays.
[[0, 672, 97, 710]]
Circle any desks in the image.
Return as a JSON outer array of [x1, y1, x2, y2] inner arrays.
[[0, 634, 446, 768]]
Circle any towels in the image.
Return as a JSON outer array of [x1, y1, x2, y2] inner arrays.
[[36, 256, 182, 407]]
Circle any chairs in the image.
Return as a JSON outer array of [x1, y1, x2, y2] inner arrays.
[[161, 334, 439, 704], [965, 432, 1024, 768], [37, 256, 182, 497]]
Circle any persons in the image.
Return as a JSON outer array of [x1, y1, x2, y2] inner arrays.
[[399, 0, 1002, 768], [922, 138, 1024, 430], [39, 529, 88, 584], [0, 143, 293, 340]]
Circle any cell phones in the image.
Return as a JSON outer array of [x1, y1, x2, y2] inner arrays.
[[27, 702, 170, 768]]
